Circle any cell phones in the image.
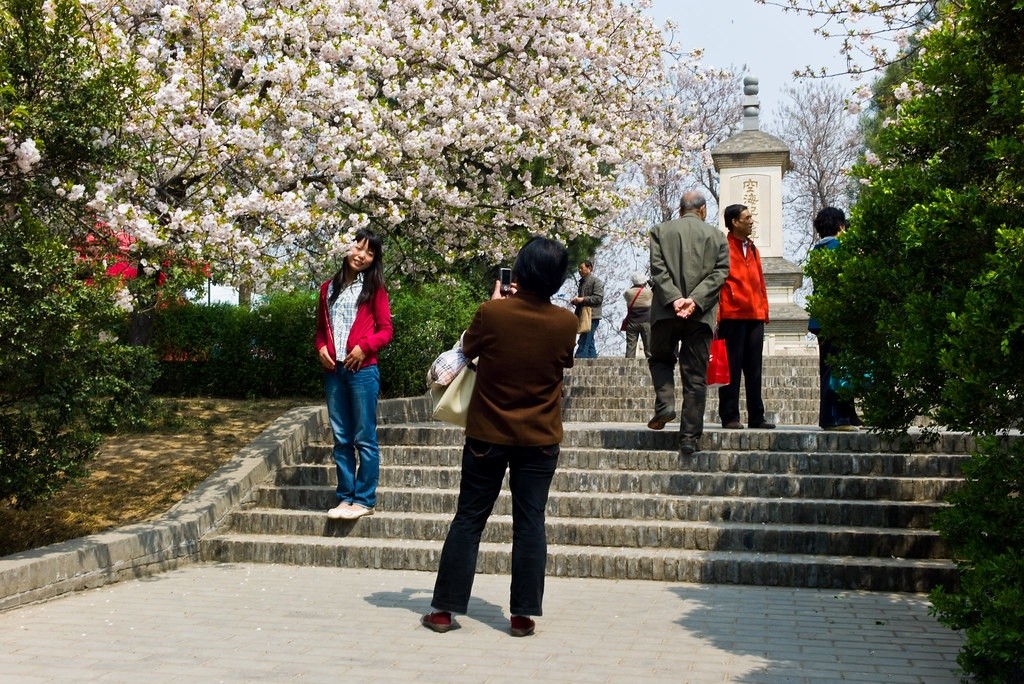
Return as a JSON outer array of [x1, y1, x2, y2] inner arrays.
[[500, 268, 511, 292]]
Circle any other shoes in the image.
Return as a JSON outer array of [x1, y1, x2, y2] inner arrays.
[[679, 439, 700, 454], [423, 611, 451, 632], [824, 423, 860, 432], [327, 502, 353, 519], [647, 405, 676, 429], [511, 615, 536, 637], [748, 421, 776, 429], [722, 422, 744, 429], [340, 503, 375, 519]]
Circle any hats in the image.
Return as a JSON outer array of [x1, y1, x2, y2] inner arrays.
[[631, 271, 650, 285]]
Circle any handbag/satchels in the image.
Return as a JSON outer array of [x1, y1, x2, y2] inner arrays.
[[425, 345, 471, 391], [429, 330, 480, 428], [576, 306, 591, 333], [620, 315, 627, 331], [704, 330, 730, 388]]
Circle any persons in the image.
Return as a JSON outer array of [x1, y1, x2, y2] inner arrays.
[[624, 273, 655, 358], [571, 260, 603, 358], [808, 208, 865, 431], [648, 191, 729, 453], [422, 236, 578, 635], [716, 203, 777, 429], [315, 228, 393, 519]]
